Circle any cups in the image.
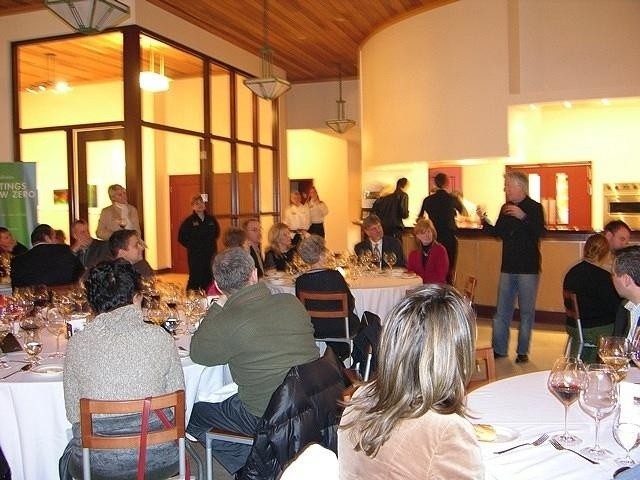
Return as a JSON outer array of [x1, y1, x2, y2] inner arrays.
[[119, 218, 127, 229]]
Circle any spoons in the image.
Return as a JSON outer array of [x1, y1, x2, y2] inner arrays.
[[0, 364, 32, 379]]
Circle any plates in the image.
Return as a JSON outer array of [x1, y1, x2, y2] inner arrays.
[[30, 364, 65, 376], [267, 278, 287, 285], [474, 423, 521, 444]]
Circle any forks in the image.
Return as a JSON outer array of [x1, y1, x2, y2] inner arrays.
[[493, 432, 549, 455]]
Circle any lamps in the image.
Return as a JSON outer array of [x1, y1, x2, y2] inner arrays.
[[139, 45, 174, 92], [44, 0, 132, 36], [242, 49, 292, 101], [26, 52, 69, 94], [326, 61, 357, 135]]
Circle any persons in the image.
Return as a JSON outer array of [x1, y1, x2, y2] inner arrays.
[[475, 171, 546, 363], [133, 250, 155, 277], [187, 245, 321, 480], [295, 233, 363, 376], [612, 244, 640, 368], [354, 178, 409, 269], [62, 256, 187, 479], [336, 283, 493, 480], [177, 193, 221, 293], [600, 220, 632, 272], [563, 233, 621, 366], [1, 224, 85, 295], [68, 184, 148, 269], [417, 173, 469, 285], [406, 219, 449, 283], [207, 186, 329, 296], [450, 190, 481, 225], [430, 185, 438, 195]]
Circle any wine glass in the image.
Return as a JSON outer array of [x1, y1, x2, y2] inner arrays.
[[347, 251, 397, 281], [0, 287, 87, 364], [147, 290, 208, 348], [547, 335, 640, 469]]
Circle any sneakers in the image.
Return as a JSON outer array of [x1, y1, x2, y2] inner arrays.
[[484, 351, 507, 360], [516, 355, 528, 363]]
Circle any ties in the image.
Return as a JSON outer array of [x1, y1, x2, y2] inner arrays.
[[374, 244, 380, 268]]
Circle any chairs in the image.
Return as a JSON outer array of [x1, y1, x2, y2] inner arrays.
[[274, 442, 340, 480], [206, 346, 345, 480], [38, 279, 80, 299], [464, 346, 495, 393], [79, 390, 187, 480], [562, 290, 599, 370], [453, 270, 477, 307], [298, 289, 353, 369]]
